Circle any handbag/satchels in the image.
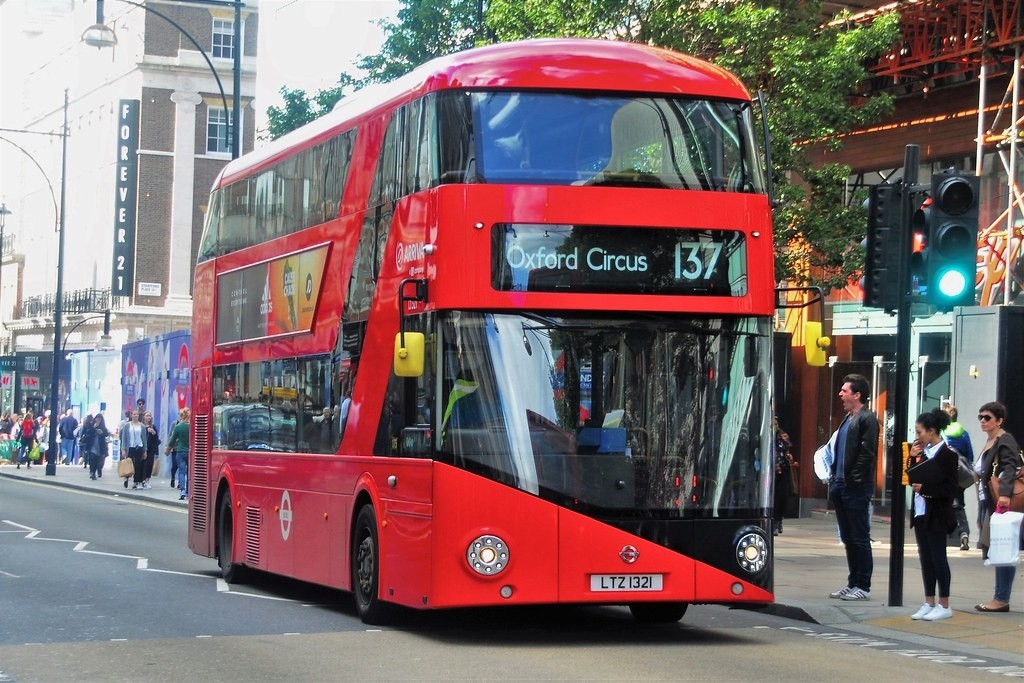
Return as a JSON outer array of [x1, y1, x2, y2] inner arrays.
[[813, 444, 834, 485], [988, 464, 1024, 514], [982, 503, 1024, 569], [28, 444, 41, 460], [79, 432, 92, 444], [118, 454, 135, 477], [948, 445, 979, 490]]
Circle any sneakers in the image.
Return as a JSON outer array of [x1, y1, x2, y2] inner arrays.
[[829, 586, 871, 601], [911, 602, 953, 622]]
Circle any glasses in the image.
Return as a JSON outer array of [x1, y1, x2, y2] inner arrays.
[[977, 415, 997, 423]]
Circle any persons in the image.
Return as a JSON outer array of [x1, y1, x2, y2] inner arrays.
[[164, 408, 190, 499], [831, 373, 881, 602], [906, 407, 958, 621], [451, 360, 503, 429], [972, 402, 1024, 612], [0, 409, 109, 480], [318, 390, 351, 450], [939, 404, 973, 551], [119, 399, 161, 490], [773, 415, 794, 536]]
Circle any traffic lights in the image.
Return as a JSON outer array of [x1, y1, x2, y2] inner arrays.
[[908, 169, 976, 306]]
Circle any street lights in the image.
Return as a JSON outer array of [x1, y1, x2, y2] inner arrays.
[[1, 89, 116, 477], [80, 0, 244, 160]]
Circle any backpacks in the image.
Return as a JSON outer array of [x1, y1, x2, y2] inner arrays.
[[23, 420, 35, 439]]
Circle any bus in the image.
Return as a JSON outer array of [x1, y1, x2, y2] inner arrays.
[[188, 39, 832, 624]]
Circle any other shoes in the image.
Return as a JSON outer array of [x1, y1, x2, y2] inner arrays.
[[61, 456, 67, 464], [17, 463, 20, 469], [123, 478, 152, 491], [27, 464, 32, 469], [170, 479, 175, 488], [774, 521, 783, 536], [90, 472, 93, 478], [973, 603, 1010, 613], [179, 495, 186, 500], [83, 462, 87, 468], [958, 533, 970, 551], [92, 477, 96, 481], [98, 474, 102, 477]]
